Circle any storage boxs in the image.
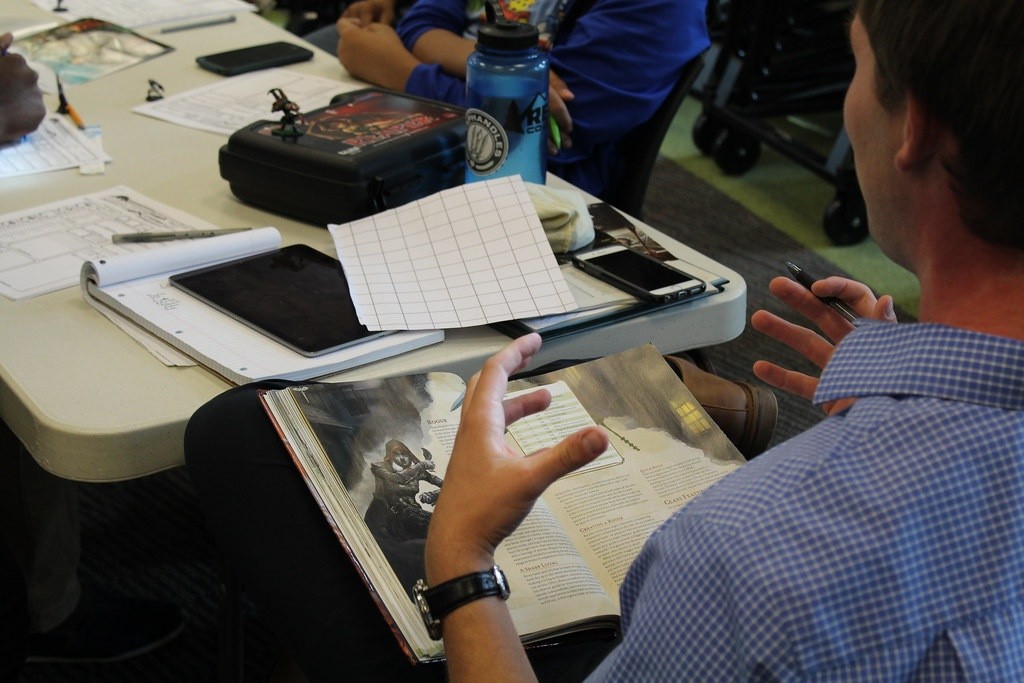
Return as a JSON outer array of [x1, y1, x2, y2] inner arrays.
[[213, 83, 469, 228]]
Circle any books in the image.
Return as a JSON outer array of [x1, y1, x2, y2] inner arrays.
[[484, 204, 729, 343], [257, 342, 748, 665], [78, 226, 444, 388]]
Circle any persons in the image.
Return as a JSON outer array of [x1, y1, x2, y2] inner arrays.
[[0, 33, 185, 664], [427, 0, 1024, 683], [303, 0, 712, 208]]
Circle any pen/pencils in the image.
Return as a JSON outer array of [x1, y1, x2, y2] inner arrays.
[[787, 260, 862, 326], [111, 226, 254, 246], [160, 16, 236, 33], [68, 102, 85, 130]]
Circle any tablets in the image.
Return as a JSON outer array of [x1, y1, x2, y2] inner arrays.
[[167, 244, 399, 358]]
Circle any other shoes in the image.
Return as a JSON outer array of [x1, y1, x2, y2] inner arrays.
[[26, 572, 186, 665]]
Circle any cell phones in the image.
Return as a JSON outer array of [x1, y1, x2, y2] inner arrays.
[[573, 243, 706, 304], [197, 41, 314, 77]]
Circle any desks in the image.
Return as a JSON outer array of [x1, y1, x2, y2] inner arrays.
[[0, 0, 747, 683]]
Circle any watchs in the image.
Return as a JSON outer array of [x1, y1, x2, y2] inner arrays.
[[411, 566, 511, 641]]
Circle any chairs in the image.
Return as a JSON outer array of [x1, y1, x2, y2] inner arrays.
[[602, 54, 704, 220]]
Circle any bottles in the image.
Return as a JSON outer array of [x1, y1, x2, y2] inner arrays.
[[463, 19, 550, 185]]
